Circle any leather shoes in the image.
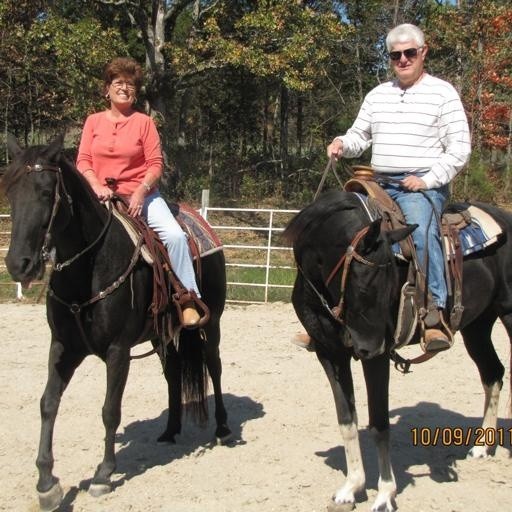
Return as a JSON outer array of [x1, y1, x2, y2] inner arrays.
[[425, 329, 449, 350], [182, 305, 200, 325], [291, 334, 315, 348]]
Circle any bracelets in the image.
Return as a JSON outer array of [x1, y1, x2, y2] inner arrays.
[[143, 182, 151, 192]]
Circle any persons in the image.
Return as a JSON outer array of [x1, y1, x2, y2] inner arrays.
[[76, 58, 202, 327], [293, 23, 472, 351]]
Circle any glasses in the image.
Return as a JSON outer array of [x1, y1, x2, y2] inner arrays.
[[111, 81, 136, 91], [389, 46, 422, 60]]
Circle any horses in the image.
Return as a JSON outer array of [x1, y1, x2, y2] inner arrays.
[[0, 129, 235, 511], [278, 188, 512, 512]]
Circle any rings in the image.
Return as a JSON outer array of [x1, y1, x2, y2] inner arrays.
[[138, 205, 141, 207], [141, 206, 143, 208]]
